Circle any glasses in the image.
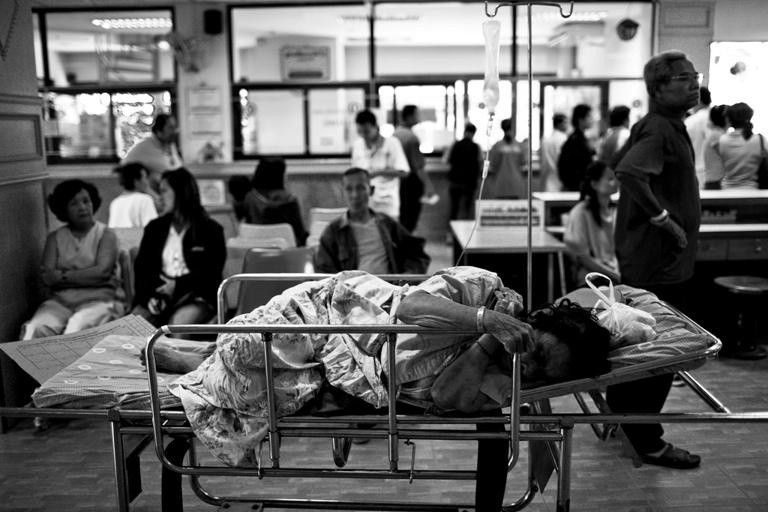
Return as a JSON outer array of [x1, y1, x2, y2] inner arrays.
[[671, 72, 705, 84]]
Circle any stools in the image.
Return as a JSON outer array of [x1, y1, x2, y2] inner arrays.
[[713, 275, 768, 360]]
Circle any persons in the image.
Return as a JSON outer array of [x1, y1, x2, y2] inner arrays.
[[390, 102, 429, 234], [605, 51, 704, 471], [129, 168, 226, 338], [229, 173, 251, 222], [139, 264, 611, 410], [18, 178, 126, 434], [107, 162, 160, 228], [441, 86, 768, 247], [120, 113, 184, 214], [311, 167, 430, 286], [561, 160, 624, 288], [243, 154, 309, 247], [350, 108, 412, 224]]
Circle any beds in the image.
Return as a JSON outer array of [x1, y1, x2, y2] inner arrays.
[[31, 273, 722, 512]]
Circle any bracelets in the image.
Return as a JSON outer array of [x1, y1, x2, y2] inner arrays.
[[61, 267, 70, 282], [474, 306, 488, 333]]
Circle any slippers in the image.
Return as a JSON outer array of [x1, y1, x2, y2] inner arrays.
[[639, 441, 701, 470]]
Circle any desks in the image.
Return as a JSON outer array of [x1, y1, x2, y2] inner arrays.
[[532, 190, 768, 263], [450, 219, 565, 302]]
